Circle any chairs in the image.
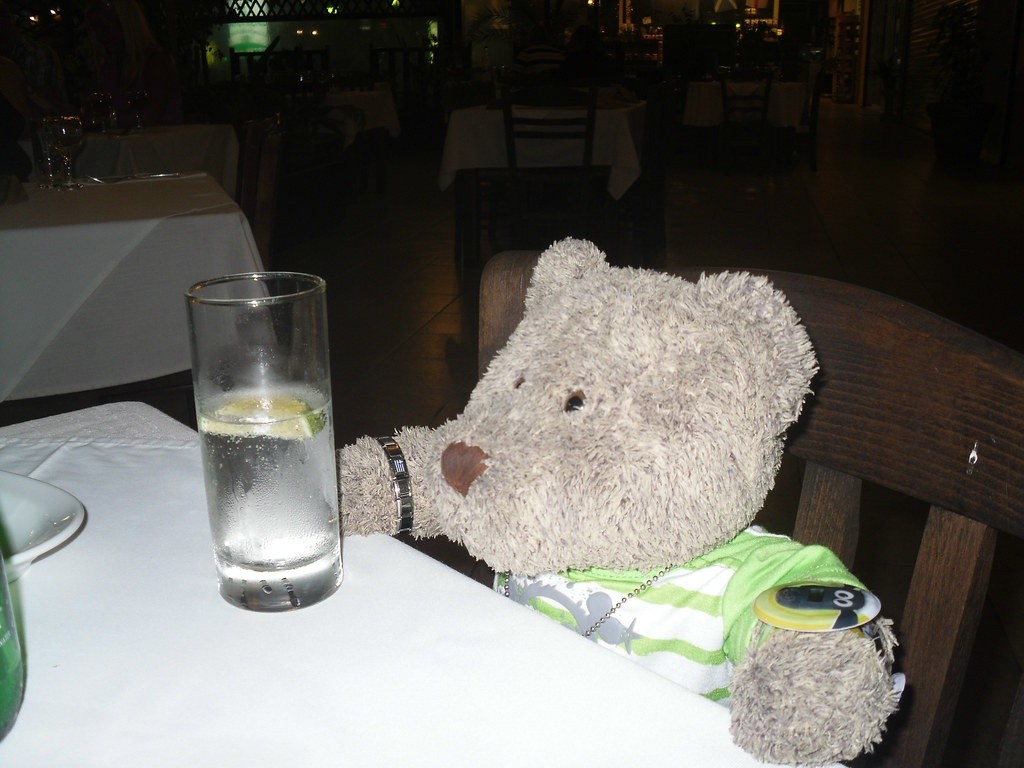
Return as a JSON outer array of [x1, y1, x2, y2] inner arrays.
[[166, 48, 1024, 768]]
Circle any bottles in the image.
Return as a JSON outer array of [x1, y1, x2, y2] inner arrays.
[[0, 551, 24, 742]]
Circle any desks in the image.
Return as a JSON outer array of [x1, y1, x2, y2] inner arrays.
[[16, 122, 239, 201], [683, 80, 807, 155], [438, 93, 646, 266], [573, 85, 632, 100], [0, 400, 845, 768], [289, 90, 400, 165], [0, 171, 275, 418]]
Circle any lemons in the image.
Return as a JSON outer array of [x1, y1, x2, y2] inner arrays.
[[197, 396, 326, 440]]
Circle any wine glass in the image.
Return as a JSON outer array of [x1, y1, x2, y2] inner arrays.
[[36, 118, 61, 191], [48, 115, 87, 191], [129, 88, 151, 130], [89, 88, 112, 136]]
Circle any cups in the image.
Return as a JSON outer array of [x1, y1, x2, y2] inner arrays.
[[185, 271, 344, 613]]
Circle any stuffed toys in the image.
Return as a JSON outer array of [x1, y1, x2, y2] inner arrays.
[[335, 237, 899, 768]]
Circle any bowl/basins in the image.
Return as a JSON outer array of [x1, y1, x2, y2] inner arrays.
[[0, 470, 85, 584]]
